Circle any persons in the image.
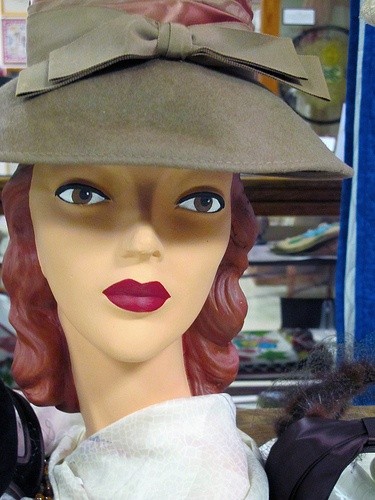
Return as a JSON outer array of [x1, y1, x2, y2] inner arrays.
[[2, 1, 273, 500]]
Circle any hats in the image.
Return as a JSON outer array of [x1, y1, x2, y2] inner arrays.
[[0, 1, 355, 182]]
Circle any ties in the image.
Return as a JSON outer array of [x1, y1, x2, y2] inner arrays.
[[266, 416, 375, 499]]
